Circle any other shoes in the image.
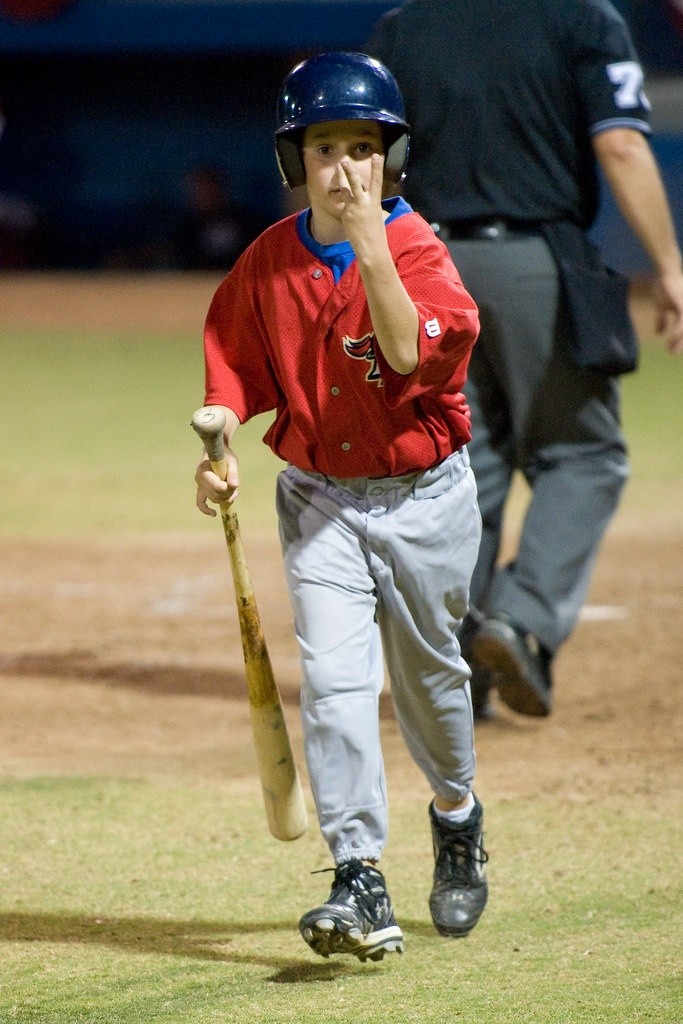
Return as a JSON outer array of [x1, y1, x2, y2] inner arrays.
[[472, 613, 553, 718], [468, 687, 489, 717]]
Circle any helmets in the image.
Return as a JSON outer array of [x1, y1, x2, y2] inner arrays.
[[271, 51, 410, 191]]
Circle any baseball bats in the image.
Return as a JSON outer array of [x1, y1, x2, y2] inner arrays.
[[191, 405, 310, 843]]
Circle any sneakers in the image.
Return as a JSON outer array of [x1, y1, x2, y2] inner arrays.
[[429, 791, 490, 936], [299, 859, 404, 963]]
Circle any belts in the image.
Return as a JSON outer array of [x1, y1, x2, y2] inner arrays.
[[439, 215, 543, 242]]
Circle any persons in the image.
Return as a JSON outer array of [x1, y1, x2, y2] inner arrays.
[[362, 0, 683, 717], [194, 52, 490, 962]]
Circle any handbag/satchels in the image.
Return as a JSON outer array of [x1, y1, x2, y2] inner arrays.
[[539, 221, 639, 377]]
